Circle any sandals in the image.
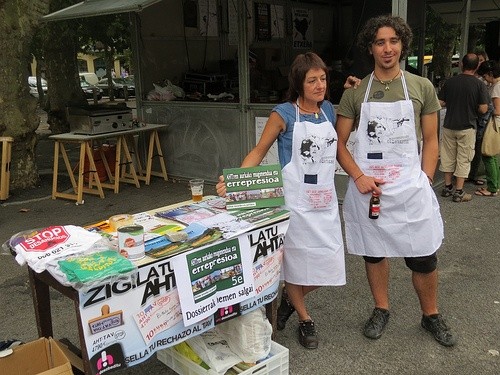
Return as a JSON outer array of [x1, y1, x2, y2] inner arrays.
[[464, 178, 469, 183], [481, 187, 500, 193], [475, 179, 485, 185], [474, 189, 497, 196]]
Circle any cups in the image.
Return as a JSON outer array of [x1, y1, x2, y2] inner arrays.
[[118, 224, 145, 259], [189, 179, 204, 201]]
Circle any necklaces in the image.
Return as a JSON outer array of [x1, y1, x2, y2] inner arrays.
[[375, 72, 399, 90], [295, 102, 318, 119]]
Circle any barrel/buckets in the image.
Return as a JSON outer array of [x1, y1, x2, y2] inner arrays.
[[84, 144, 117, 183]]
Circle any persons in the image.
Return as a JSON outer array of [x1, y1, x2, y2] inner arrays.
[[335, 16, 456, 346], [216, 52, 361, 349], [465, 51, 489, 183], [438, 53, 489, 201], [112, 70, 116, 78], [122, 70, 128, 78], [475, 60, 500, 196]]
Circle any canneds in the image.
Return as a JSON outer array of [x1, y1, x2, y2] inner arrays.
[[117, 225, 145, 260]]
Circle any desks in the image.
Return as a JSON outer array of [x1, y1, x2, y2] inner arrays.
[[49, 121, 168, 204], [8, 194, 290, 375]]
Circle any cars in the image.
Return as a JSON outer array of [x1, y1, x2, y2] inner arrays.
[[96, 77, 136, 99], [78, 80, 104, 101], [27, 76, 48, 96]]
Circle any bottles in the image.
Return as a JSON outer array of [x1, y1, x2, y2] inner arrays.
[[369, 182, 380, 219]]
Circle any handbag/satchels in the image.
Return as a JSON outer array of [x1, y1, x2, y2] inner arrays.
[[148, 79, 185, 102], [218, 305, 272, 363], [481, 115, 500, 156]]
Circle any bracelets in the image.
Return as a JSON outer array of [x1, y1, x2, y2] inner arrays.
[[354, 174, 364, 183]]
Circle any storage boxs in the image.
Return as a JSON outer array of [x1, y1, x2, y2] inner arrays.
[[0, 336, 85, 375], [156, 338, 290, 375], [184, 72, 229, 94]]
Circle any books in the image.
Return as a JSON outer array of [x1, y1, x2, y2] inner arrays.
[[87, 196, 290, 260]]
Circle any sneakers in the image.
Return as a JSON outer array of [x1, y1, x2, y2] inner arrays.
[[421, 313, 456, 346], [297, 320, 318, 349], [363, 309, 390, 339], [453, 191, 472, 202], [441, 185, 455, 197], [277, 284, 297, 331]]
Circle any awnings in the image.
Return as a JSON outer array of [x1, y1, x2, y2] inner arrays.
[[43, 0, 161, 22]]
